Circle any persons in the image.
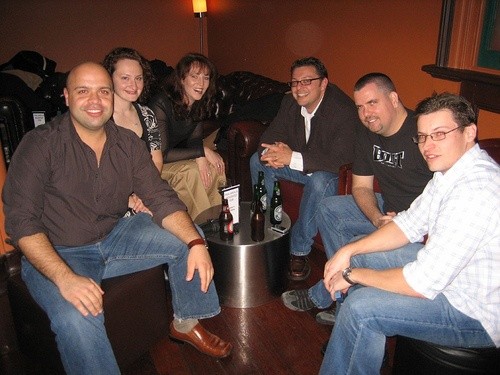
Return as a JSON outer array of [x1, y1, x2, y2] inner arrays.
[[148, 53, 247, 202], [318, 90, 500, 375], [1, 61, 233, 375], [281, 73, 436, 326], [249, 56, 360, 281], [102, 47, 225, 226]]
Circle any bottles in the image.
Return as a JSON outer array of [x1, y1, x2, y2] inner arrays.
[[219, 199, 234, 241], [270, 181, 283, 225], [250, 200, 265, 242], [257, 171, 268, 212], [250, 183, 264, 217]]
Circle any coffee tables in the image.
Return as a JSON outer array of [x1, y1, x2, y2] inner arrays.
[[194, 203, 290, 308]]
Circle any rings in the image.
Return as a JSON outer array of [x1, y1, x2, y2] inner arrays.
[[207, 174, 209, 176], [218, 165, 220, 167]]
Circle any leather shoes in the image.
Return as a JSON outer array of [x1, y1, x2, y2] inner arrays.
[[168, 320, 234, 359], [289, 254, 313, 282]]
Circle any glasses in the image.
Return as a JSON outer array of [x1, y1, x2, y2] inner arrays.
[[412, 125, 461, 144], [288, 77, 321, 87]]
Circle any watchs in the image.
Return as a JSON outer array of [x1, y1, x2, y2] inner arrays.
[[342, 267, 354, 284], [188, 238, 209, 249]]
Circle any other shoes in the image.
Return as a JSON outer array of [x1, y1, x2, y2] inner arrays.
[[281, 289, 317, 312], [321, 340, 386, 369], [316, 308, 340, 327]]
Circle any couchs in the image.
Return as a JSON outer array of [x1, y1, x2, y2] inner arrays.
[[35, 60, 285, 163], [229, 108, 379, 250]]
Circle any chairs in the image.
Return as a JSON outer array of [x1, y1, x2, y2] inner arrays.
[[384, 137, 500, 374], [0, 99, 171, 375]]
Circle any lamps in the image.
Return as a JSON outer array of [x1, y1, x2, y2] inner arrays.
[[192, 0, 208, 53]]
